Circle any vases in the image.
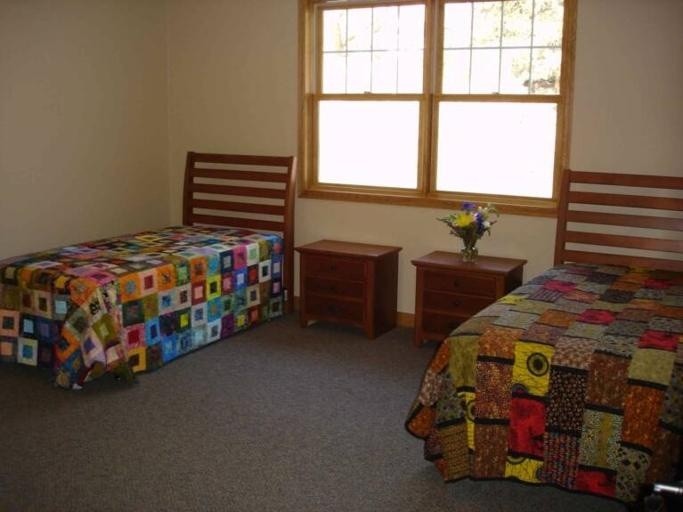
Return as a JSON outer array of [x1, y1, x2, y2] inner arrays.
[[460, 239, 478, 262]]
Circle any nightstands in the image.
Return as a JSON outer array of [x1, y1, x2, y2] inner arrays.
[[411, 250, 528, 345], [294, 239, 403, 339]]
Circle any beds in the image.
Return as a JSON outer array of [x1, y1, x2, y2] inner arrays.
[[0, 150, 297, 389], [424, 167, 683, 511]]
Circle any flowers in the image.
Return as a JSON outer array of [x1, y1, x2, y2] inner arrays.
[[436, 199, 501, 262]]
